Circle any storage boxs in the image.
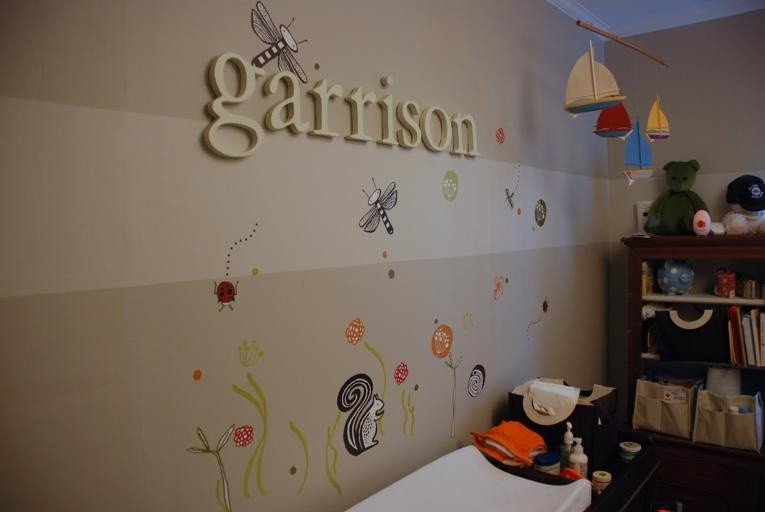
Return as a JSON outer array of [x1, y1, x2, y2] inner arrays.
[[654, 303, 730, 363], [508, 377, 618, 477], [632, 375, 701, 439], [692, 382, 765, 454]]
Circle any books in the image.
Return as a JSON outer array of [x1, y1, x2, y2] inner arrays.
[[726, 306, 765, 368]]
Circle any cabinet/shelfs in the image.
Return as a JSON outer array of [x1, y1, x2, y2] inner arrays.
[[618, 231, 765, 512]]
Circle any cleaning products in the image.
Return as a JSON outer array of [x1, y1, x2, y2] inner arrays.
[[561, 422, 573, 468], [569, 437, 587, 476]]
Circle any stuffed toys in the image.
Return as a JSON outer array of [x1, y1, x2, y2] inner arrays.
[[693, 173, 765, 234], [644, 158, 712, 235]]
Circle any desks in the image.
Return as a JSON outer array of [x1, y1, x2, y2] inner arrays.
[[484, 440, 661, 512]]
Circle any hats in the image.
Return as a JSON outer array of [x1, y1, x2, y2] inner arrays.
[[727, 175, 765, 211]]
